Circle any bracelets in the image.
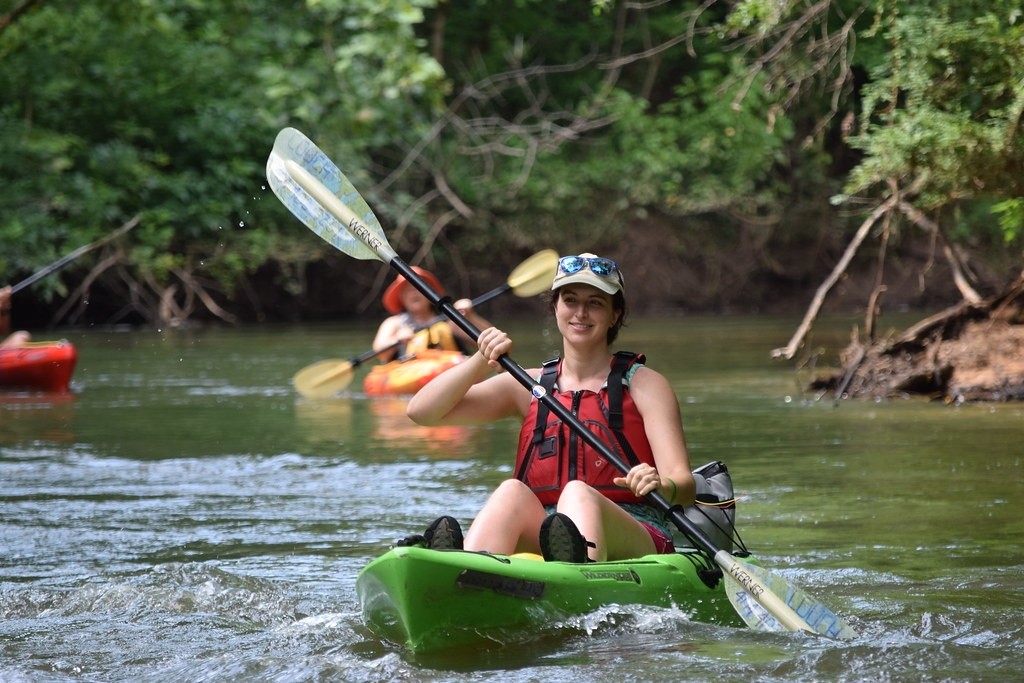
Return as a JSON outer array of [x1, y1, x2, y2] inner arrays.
[[662, 476, 677, 505]]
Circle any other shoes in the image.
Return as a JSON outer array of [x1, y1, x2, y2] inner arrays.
[[539, 512, 589, 566], [424, 515, 464, 552]]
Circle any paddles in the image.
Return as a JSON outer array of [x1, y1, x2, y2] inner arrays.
[[266, 126, 860, 642], [293, 248, 560, 401], [10, 210, 149, 295]]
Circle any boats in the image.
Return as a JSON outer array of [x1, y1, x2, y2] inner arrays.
[[352, 550, 765, 656], [0, 339, 78, 390], [363, 349, 468, 398]]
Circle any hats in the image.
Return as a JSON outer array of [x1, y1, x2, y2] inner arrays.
[[552, 250, 625, 299], [382, 265, 448, 314]]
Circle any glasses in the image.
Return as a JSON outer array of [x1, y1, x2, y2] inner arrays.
[[556, 256, 624, 288]]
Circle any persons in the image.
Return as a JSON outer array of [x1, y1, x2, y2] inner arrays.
[[0, 276, 31, 343], [372, 265, 494, 363], [407, 253, 696, 563]]
